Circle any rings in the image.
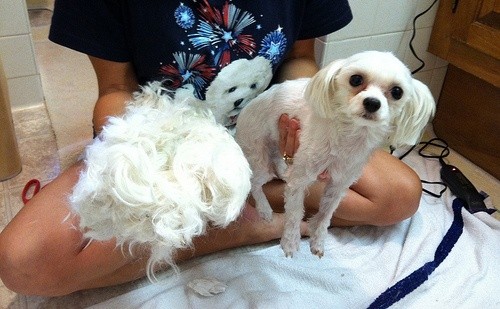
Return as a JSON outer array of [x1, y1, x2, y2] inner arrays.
[[282, 151, 295, 163]]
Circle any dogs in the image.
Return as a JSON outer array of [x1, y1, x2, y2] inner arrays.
[[234, 51, 436, 260]]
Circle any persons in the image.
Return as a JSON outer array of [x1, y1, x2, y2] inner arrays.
[[0, 0, 423, 300]]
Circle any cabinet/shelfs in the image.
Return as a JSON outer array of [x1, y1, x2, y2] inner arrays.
[[425, 1, 500, 89]]
